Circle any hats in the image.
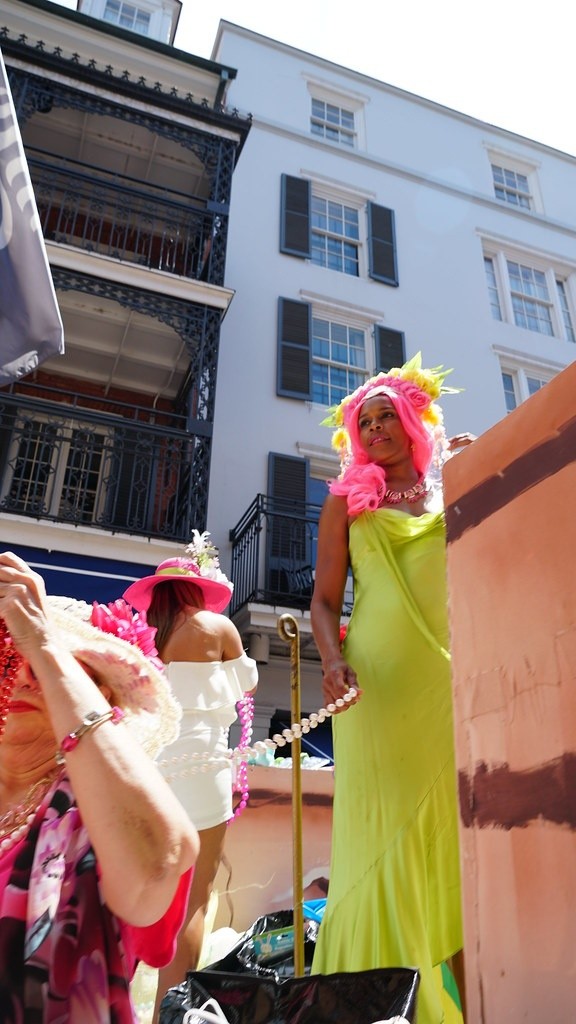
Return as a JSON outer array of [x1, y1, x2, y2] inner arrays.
[[122, 557, 232, 615], [44, 596, 181, 761]]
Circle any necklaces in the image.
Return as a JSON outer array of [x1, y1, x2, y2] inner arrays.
[[0, 760, 65, 841], [385, 476, 425, 506]]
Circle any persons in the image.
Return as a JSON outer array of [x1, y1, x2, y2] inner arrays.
[[0, 549, 200, 1024], [311, 383, 481, 1024], [121, 557, 262, 1024]]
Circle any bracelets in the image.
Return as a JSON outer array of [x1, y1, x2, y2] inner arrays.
[[57, 706, 124, 766]]
